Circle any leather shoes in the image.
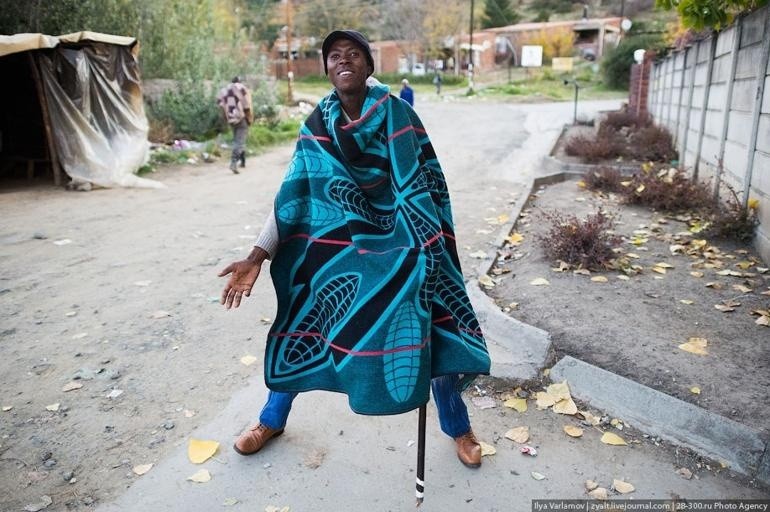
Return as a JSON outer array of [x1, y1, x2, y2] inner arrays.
[[233, 421, 285, 456], [455, 428, 483, 471]]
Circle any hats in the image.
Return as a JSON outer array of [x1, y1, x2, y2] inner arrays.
[[319, 28, 375, 79]]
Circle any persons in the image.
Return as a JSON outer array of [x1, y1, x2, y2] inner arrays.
[[399, 78, 414, 107], [217, 76, 253, 174], [435, 73, 442, 94], [217, 30, 489, 469]]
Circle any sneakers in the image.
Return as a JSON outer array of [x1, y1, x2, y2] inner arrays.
[[229, 156, 246, 174]]
